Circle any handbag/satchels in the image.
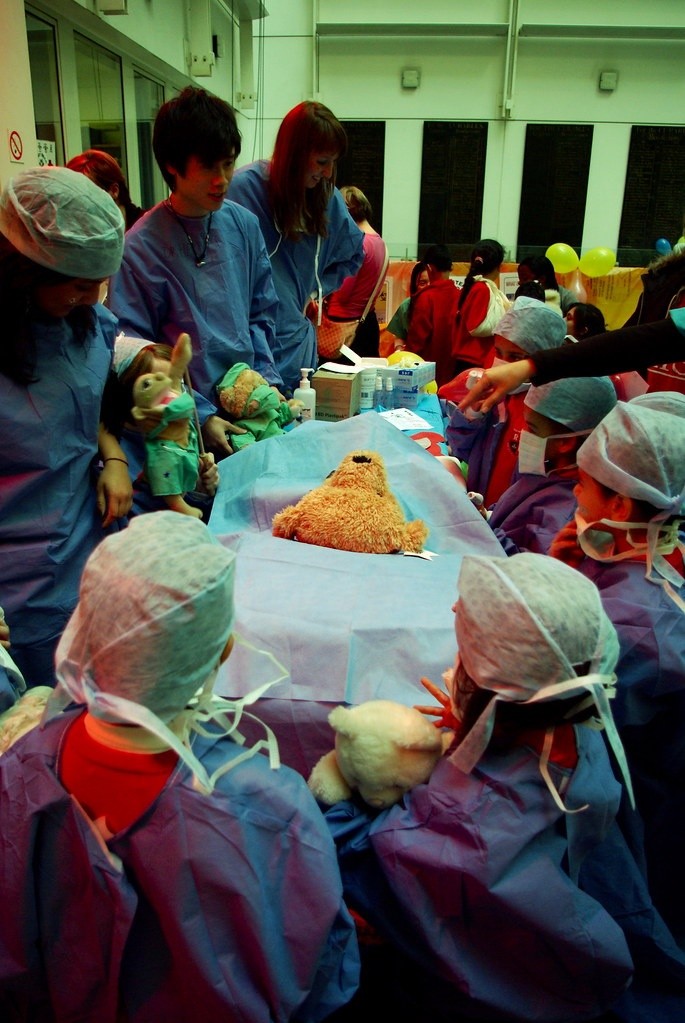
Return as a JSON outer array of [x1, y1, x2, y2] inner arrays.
[[310, 307, 360, 360]]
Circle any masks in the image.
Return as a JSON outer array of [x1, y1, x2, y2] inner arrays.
[[492, 357, 533, 395], [517, 429, 599, 478], [572, 508, 677, 562], [441, 658, 463, 723]]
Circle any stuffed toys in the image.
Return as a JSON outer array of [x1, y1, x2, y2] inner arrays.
[[306, 699, 454, 806], [216, 362, 305, 449], [132, 333, 203, 519], [272, 449, 430, 554], [0, 686, 55, 757]]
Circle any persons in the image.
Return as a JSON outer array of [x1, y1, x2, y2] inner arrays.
[[328, 186, 389, 364], [110, 84, 284, 458], [0, 167, 133, 693], [387, 239, 685, 553], [66, 149, 147, 232], [115, 344, 220, 522], [225, 100, 366, 388], [317, 551, 685, 1023], [545, 400, 685, 940], [0, 511, 364, 1023]]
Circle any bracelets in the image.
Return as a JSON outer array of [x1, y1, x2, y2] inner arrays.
[[103, 458, 129, 468], [395, 344, 402, 350]]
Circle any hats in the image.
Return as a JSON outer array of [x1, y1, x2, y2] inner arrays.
[[109, 331, 156, 378]]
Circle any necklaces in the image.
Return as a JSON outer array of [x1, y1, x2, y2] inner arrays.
[[167, 197, 213, 267]]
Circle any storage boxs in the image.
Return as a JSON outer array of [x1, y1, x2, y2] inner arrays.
[[312, 370, 362, 423]]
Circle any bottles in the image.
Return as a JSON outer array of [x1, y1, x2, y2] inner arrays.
[[373, 376, 384, 413], [384, 377, 395, 411]]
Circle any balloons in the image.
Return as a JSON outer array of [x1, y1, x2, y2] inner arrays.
[[579, 246, 616, 277], [546, 243, 579, 273], [388, 351, 438, 393], [439, 368, 485, 402], [656, 239, 671, 255], [568, 270, 587, 304]]
